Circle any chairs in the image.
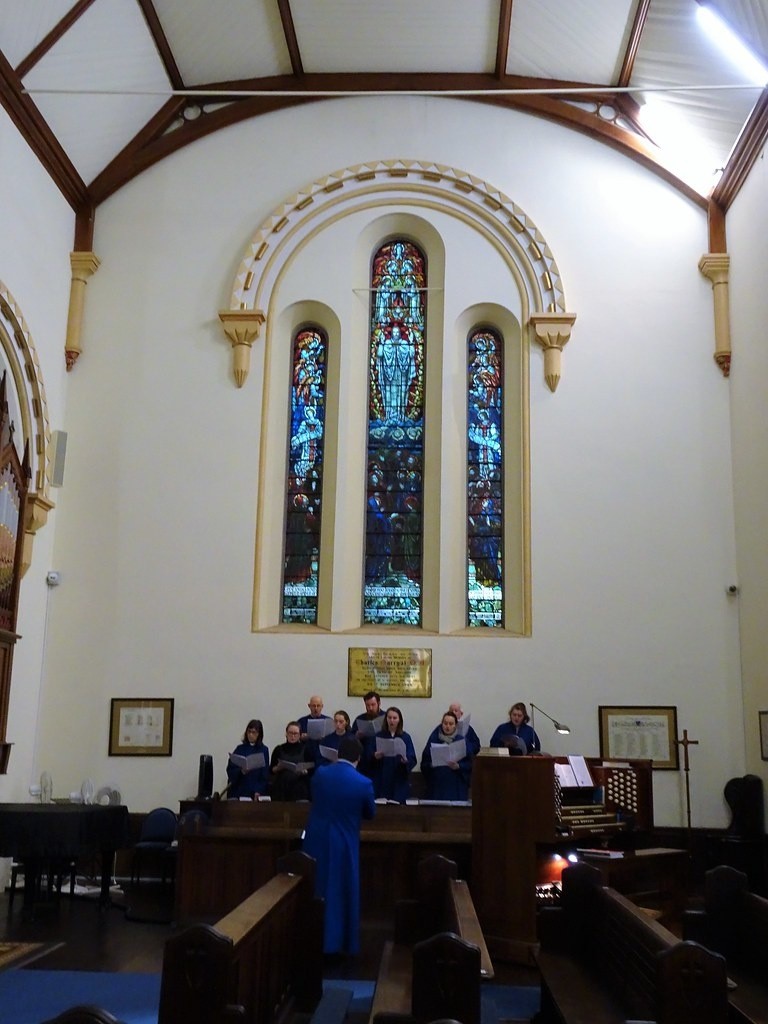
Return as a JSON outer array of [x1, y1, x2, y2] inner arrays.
[[9, 861, 75, 906], [125, 808, 176, 924]]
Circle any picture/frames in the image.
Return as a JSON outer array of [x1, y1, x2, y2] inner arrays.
[[107, 697, 175, 757], [599, 706, 681, 772]]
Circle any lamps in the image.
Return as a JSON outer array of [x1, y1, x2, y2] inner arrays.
[[529, 703, 571, 756]]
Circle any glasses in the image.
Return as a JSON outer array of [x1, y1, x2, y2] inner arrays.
[[246, 729, 258, 736], [286, 732, 300, 737]]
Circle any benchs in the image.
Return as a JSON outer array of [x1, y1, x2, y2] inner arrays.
[[156, 848, 328, 1023], [369, 855, 768, 1024]]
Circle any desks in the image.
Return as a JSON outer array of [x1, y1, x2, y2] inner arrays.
[[0, 803, 139, 934]]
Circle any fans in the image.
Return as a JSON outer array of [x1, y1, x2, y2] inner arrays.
[[69, 779, 93, 805], [94, 782, 123, 807], [30, 771, 52, 804]]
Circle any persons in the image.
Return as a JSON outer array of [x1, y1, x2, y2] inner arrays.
[[296, 695, 337, 754], [420, 703, 481, 801], [315, 710, 353, 763], [352, 691, 386, 743], [302, 736, 375, 965], [363, 706, 417, 804], [269, 721, 315, 801], [490, 703, 541, 751], [227, 720, 271, 801]]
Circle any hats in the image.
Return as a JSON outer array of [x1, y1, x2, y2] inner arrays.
[[339, 736, 363, 754]]
